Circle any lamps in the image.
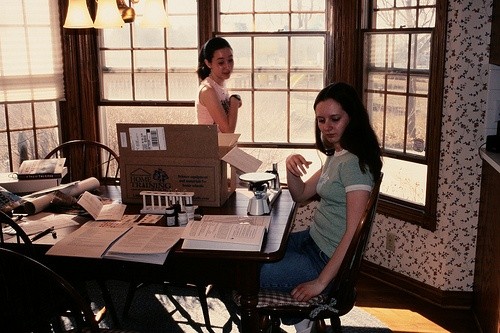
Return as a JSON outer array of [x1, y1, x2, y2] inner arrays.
[[63, 0, 174, 28]]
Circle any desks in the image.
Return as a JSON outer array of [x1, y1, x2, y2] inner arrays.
[[0, 185, 298, 333]]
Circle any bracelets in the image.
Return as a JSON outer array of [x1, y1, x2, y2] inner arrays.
[[229, 94, 240, 101]]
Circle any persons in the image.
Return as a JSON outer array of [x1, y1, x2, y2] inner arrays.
[[223, 83, 384, 333], [195, 36, 242, 133]]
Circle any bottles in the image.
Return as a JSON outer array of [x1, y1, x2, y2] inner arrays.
[[164, 208, 176, 226], [186, 204, 194, 219], [178, 211, 188, 225], [270, 163, 280, 188]]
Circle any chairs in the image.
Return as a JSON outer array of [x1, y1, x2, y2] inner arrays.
[[232, 172, 384, 333], [0, 140, 120, 333]]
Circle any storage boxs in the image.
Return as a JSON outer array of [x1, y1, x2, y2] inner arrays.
[[116, 123, 263, 207]]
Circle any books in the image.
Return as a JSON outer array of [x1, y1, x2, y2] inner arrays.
[[17, 158, 272, 266]]
